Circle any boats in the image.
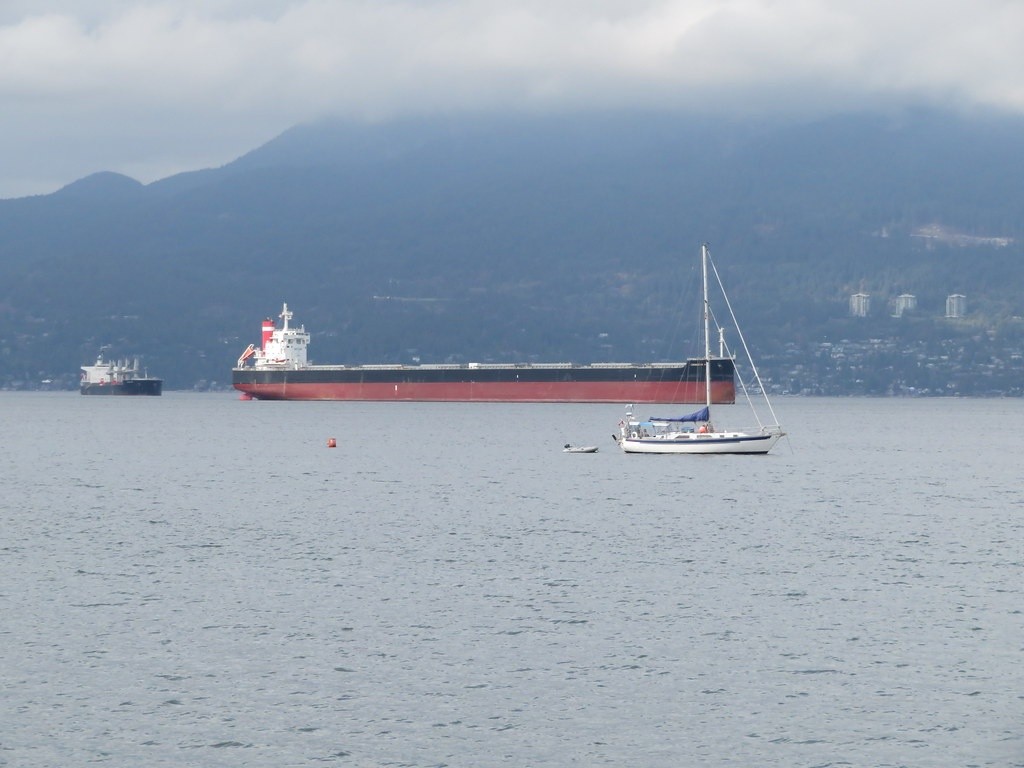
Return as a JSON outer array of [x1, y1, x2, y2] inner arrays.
[[79, 345, 165, 397], [563, 445, 599, 453]]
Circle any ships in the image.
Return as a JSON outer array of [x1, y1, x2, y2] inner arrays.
[[232, 303, 737, 405]]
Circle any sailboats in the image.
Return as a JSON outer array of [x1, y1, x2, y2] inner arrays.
[[612, 240, 788, 455]]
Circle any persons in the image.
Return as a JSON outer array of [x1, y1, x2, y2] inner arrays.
[[698, 421, 714, 433], [637, 425, 657, 438]]
